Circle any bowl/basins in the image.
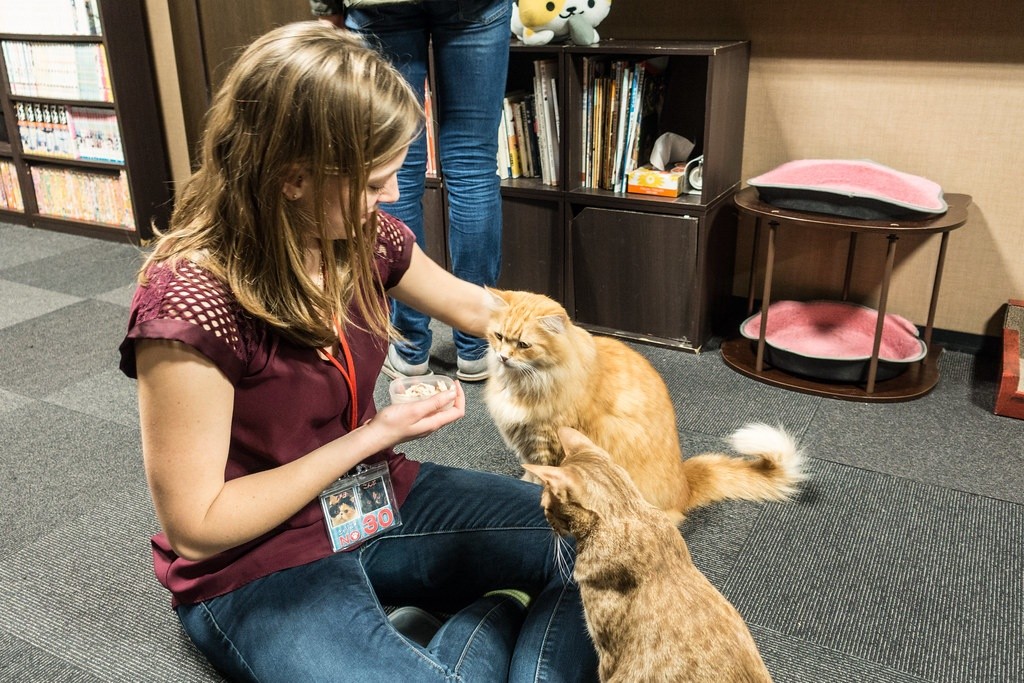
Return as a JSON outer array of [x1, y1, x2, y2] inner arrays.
[[388, 375, 455, 412]]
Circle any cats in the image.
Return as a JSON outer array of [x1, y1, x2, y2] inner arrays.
[[521, 426, 776, 683], [328, 479, 385, 527], [482, 282, 809, 527]]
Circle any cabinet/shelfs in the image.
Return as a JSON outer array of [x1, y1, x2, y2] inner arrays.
[[721, 186, 972, 404], [0, 0, 175, 246], [422, 37, 749, 353]]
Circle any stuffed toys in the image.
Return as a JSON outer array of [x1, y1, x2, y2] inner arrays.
[[510, 0, 611, 45]]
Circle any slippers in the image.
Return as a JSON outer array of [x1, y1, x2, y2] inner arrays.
[[381, 343, 434, 380], [456, 367, 489, 382]]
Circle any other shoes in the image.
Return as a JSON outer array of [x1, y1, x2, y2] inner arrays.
[[387, 606, 443, 648]]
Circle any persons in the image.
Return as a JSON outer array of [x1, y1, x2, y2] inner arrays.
[[312, 0, 513, 381], [119, 19, 600, 683]]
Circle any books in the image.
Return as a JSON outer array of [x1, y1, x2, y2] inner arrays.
[[424, 57, 674, 194], [0, 1, 136, 229]]
